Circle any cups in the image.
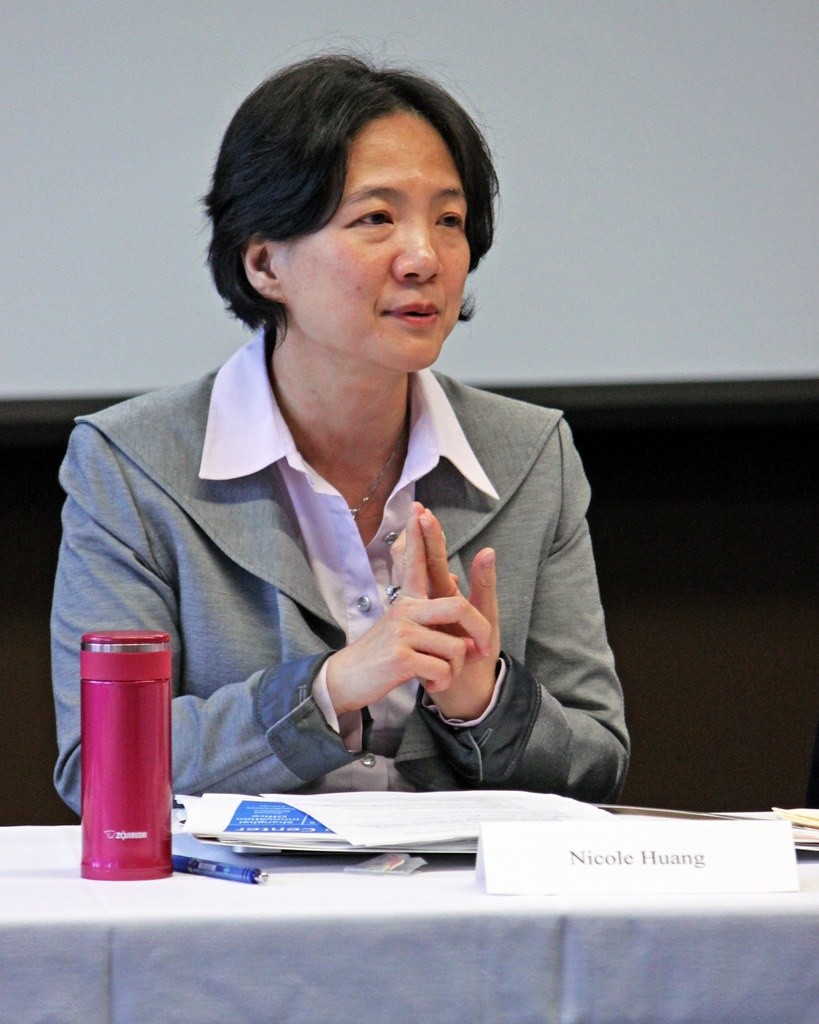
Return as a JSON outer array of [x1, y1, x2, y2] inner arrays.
[[79, 630, 173, 882]]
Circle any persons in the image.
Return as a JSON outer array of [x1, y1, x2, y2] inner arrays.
[[49, 46, 634, 822]]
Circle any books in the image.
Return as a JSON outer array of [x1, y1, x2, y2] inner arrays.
[[174, 788, 614, 852]]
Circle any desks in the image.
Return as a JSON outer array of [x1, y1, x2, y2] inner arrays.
[[1, 825, 819, 1022]]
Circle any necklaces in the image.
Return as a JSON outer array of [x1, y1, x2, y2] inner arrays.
[[349, 427, 404, 520]]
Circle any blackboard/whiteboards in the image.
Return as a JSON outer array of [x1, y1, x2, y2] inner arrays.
[[1, 1, 819, 424]]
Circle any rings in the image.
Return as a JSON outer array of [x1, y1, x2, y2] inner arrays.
[[386, 583, 400, 603]]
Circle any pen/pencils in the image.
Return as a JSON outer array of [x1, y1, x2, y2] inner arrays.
[[171, 854, 269, 885]]
